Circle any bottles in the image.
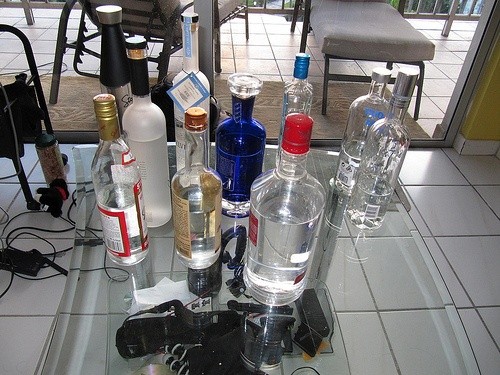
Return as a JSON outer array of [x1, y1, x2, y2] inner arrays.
[[277, 53, 314, 154], [90, 94, 149, 266], [214, 73, 266, 219], [122, 37, 172, 228], [335, 67, 419, 230], [170, 107, 223, 270], [172, 12, 210, 172], [95, 5, 133, 137], [243, 113, 326, 307]]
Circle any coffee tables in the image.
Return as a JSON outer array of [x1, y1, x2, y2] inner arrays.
[[41, 143, 468, 375]]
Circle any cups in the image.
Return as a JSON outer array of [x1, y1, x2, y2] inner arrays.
[[35, 133, 65, 184]]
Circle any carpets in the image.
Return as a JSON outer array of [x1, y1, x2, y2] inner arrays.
[[0, 74, 432, 141]]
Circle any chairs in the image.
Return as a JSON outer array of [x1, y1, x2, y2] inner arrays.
[[0, 24, 68, 211], [49, 0, 249, 104], [291, 0, 435, 120]]
